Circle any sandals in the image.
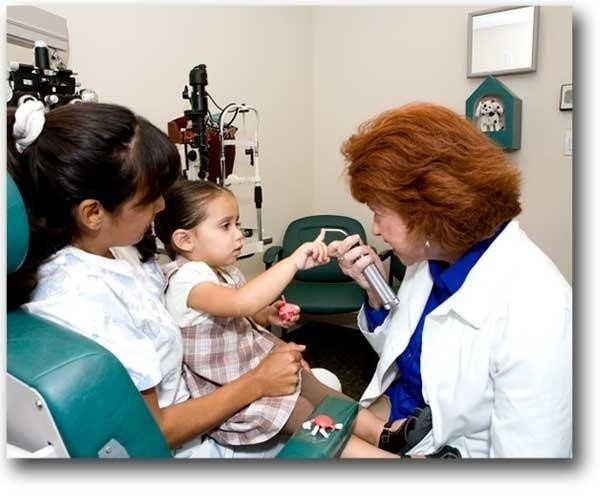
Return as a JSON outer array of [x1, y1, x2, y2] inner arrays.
[[401, 445, 461, 458], [378, 405, 432, 454]]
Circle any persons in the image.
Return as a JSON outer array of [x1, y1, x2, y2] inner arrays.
[[7, 97, 342, 458], [327, 102, 572, 459], [132, 181, 462, 458]]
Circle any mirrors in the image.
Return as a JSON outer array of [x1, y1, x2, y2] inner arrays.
[[466, 6, 539, 76]]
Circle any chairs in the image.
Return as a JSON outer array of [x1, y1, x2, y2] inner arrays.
[[264, 215, 374, 341], [6, 174, 361, 459], [382, 249, 406, 292]]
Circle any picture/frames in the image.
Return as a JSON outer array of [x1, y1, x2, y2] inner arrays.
[[559, 84, 573, 110]]
